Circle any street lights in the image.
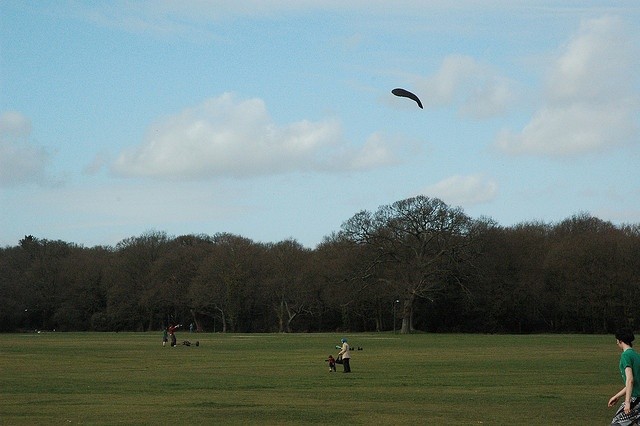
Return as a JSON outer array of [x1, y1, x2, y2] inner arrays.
[[394, 300, 400, 335]]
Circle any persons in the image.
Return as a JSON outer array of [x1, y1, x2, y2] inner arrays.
[[190, 323, 193, 333], [328, 355, 336, 372], [168, 324, 177, 347], [162, 326, 168, 348], [607, 327, 640, 426], [193, 323, 197, 333], [338, 338, 351, 373]]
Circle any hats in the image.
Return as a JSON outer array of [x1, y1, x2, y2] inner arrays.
[[341, 338, 347, 343]]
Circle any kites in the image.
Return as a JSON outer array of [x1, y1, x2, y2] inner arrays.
[[391, 88, 423, 109]]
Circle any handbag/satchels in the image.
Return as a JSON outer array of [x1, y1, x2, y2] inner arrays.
[[336, 355, 343, 364]]
